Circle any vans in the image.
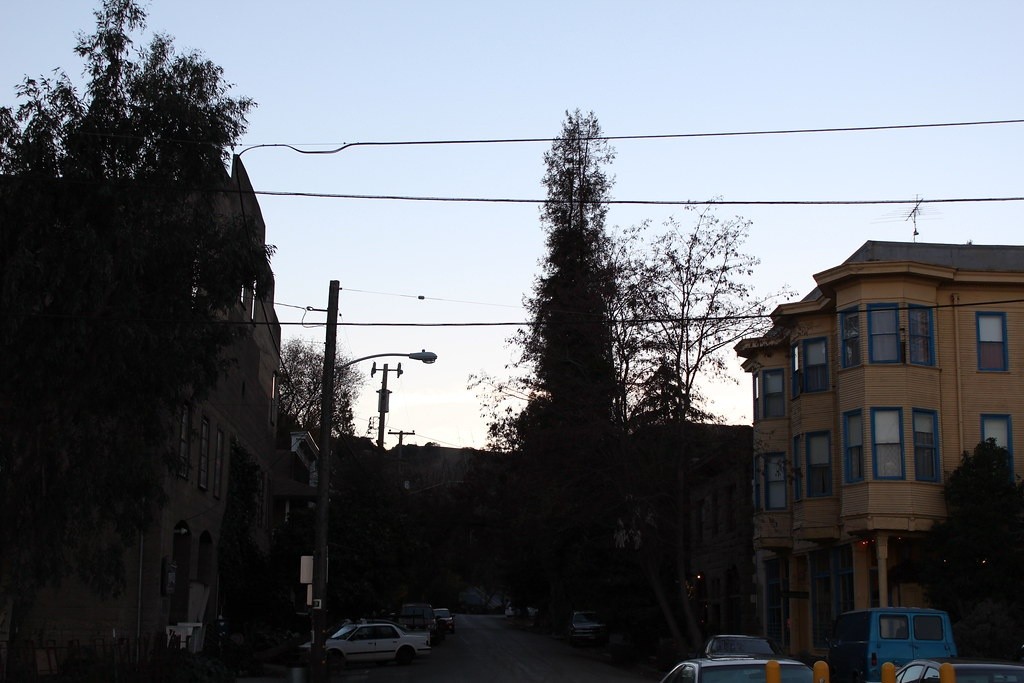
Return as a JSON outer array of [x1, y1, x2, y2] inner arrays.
[[825, 607, 956, 683]]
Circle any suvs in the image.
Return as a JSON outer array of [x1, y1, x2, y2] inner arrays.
[[391, 602, 438, 642]]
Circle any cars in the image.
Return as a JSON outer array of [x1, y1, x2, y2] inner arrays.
[[688, 635, 790, 658], [564, 610, 608, 645], [892, 657, 1023, 683], [656, 657, 818, 682], [433, 607, 457, 634], [505, 602, 539, 617], [295, 619, 432, 668]]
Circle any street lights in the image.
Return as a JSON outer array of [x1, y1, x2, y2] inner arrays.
[[306, 350, 438, 680]]
